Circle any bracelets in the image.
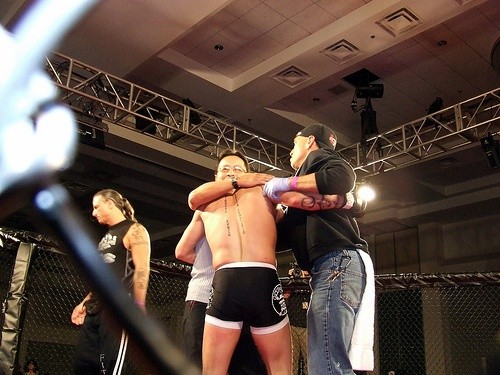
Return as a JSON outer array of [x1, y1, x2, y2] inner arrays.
[[289, 177, 298, 190]]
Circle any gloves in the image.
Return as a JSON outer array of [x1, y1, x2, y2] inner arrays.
[[341, 192, 366, 216], [262, 178, 289, 203]]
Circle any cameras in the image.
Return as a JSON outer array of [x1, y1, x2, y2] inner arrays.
[[291, 264, 302, 278]]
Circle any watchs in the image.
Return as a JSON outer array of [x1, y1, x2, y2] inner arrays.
[[230, 176, 240, 190]]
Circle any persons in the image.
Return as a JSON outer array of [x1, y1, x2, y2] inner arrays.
[[262, 125, 375, 375], [175, 184, 367, 375], [70, 189, 150, 375], [174, 149, 277, 375], [23, 357, 39, 375]]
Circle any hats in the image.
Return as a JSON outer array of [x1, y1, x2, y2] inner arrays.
[[295, 123, 337, 150]]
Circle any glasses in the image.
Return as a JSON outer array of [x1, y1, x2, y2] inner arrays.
[[216, 167, 247, 173]]
